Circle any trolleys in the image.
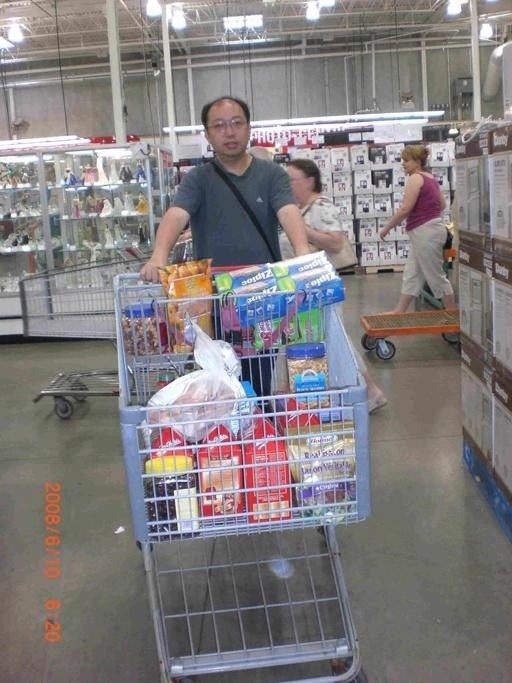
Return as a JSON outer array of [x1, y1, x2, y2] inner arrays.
[[356, 302, 464, 361], [18, 236, 196, 420], [113, 269, 370, 678]]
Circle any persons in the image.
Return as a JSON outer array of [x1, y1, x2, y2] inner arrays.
[[137, 94, 310, 406], [378, 144, 457, 313], [279, 160, 389, 413]]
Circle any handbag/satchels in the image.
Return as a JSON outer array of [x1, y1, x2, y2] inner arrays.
[[443, 229, 453, 250], [328, 238, 358, 269]]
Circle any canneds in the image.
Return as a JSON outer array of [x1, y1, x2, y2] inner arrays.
[[286, 342, 330, 410]]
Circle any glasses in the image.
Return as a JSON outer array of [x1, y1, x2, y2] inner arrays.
[[211, 119, 243, 130]]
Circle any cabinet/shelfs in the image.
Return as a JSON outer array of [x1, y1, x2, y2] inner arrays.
[[0, 142, 174, 320]]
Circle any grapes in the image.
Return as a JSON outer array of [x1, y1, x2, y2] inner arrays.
[[145, 476, 198, 537]]
